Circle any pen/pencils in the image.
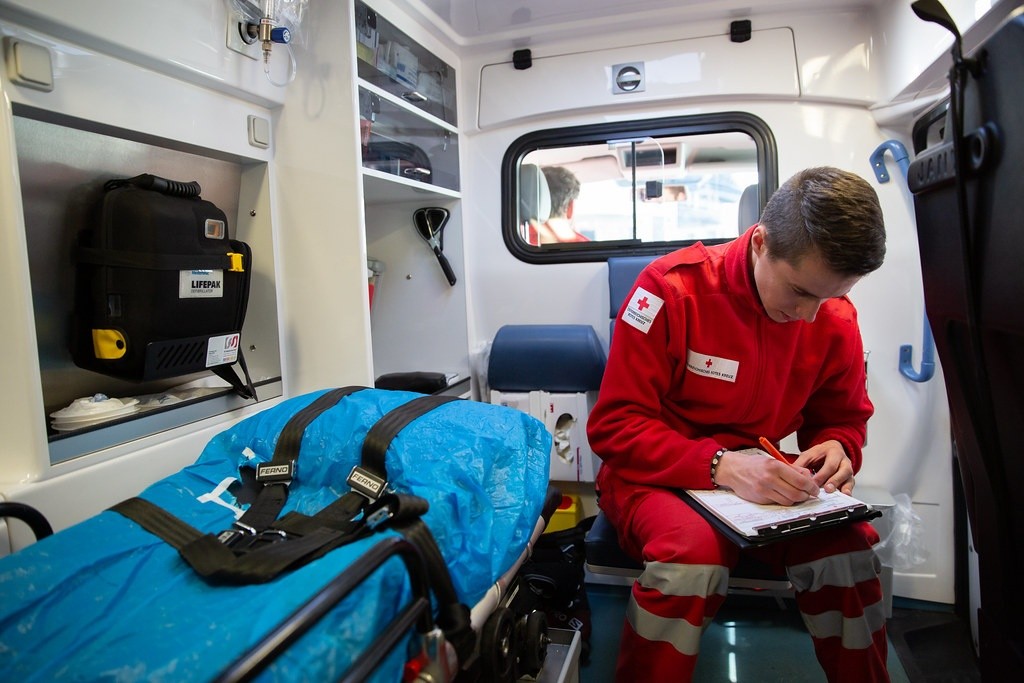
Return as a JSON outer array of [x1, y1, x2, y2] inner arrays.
[[758, 436, 821, 500]]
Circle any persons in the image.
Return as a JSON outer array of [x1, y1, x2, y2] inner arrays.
[[585, 167, 886, 681], [529, 166, 591, 247], [662, 186, 686, 203]]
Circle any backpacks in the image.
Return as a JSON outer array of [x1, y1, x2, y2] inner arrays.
[[70, 173, 253, 384]]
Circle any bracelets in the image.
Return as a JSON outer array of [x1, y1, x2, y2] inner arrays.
[[710, 447, 728, 490]]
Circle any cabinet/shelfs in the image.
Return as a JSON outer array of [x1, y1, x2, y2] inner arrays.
[[272, 0, 465, 405]]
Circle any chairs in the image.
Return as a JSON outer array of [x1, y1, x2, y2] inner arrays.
[[582, 254, 798, 605]]
